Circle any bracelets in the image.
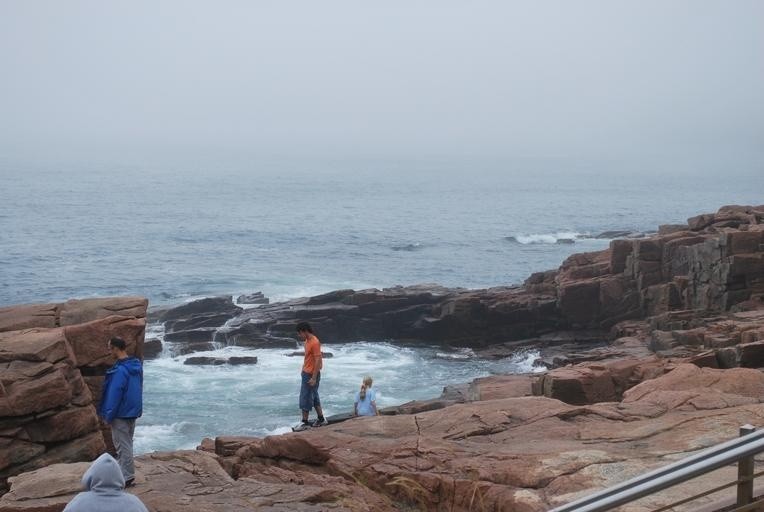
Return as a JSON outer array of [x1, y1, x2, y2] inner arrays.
[[311, 378, 317, 380]]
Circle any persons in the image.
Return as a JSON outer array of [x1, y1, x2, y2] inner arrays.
[[97, 336, 143, 488], [291, 322, 328, 432], [352, 376, 380, 417], [61, 452, 150, 512]]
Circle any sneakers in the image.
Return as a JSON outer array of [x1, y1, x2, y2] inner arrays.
[[126, 477, 134, 485], [313, 417, 329, 426], [292, 421, 309, 432]]
